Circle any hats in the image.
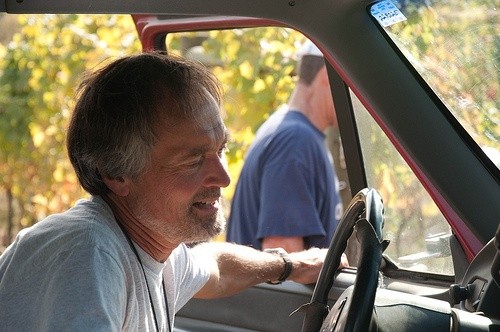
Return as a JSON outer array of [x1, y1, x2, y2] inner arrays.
[[300, 39, 324, 59]]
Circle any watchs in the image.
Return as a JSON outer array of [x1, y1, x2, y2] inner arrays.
[[262, 247, 291, 285]]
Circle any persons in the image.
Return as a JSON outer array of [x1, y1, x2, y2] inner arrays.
[[1, 52, 347, 332], [225, 44, 342, 253]]
